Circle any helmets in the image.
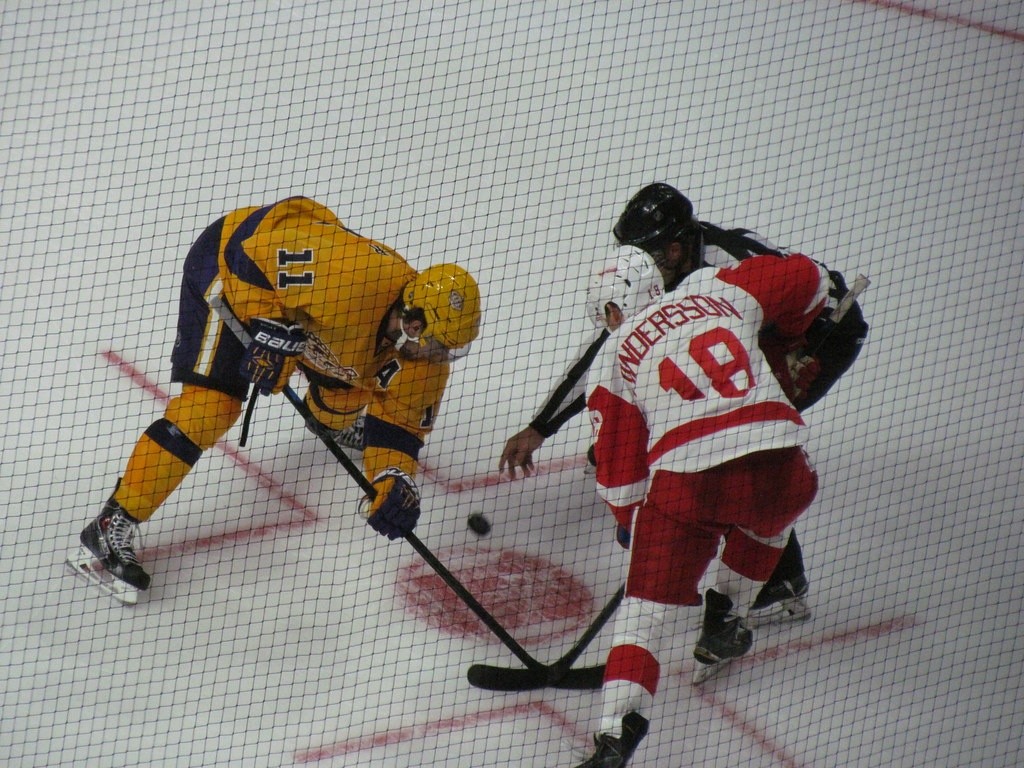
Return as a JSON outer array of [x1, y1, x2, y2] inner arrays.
[[613, 182, 692, 251], [403, 262, 481, 350], [585, 244, 666, 328]]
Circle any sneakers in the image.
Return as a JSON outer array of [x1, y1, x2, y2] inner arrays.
[[692, 588, 753, 685], [305, 415, 364, 452], [745, 563, 810, 628], [65, 477, 150, 604], [575, 712, 650, 768]]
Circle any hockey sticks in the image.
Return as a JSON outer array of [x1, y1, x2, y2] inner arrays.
[[467, 273, 874, 693], [281, 385, 606, 689]]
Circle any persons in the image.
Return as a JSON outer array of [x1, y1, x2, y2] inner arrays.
[[65, 196, 481, 607], [571, 245, 831, 768], [497, 183, 869, 626]]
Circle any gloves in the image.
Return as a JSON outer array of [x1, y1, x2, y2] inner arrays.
[[238, 317, 305, 396], [358, 467, 421, 540], [784, 336, 821, 402], [616, 524, 631, 551]]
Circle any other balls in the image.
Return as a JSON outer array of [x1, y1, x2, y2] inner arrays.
[[467, 515, 491, 534]]
